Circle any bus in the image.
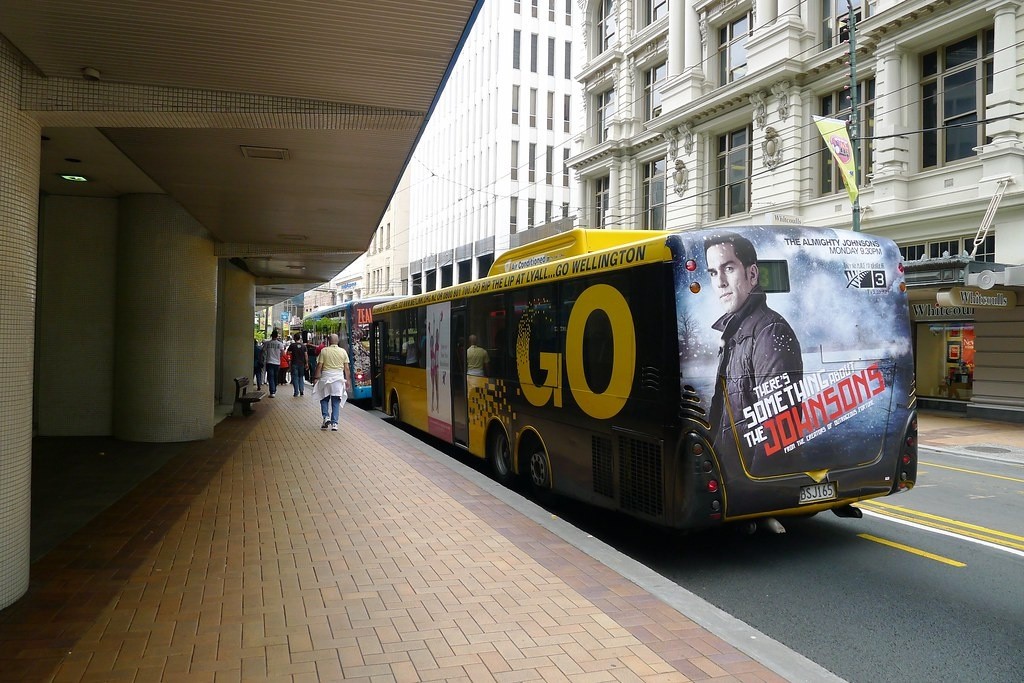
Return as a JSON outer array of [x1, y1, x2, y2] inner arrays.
[[370, 224, 918, 534], [301, 296, 414, 400]]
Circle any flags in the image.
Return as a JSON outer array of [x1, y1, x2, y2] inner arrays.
[[813, 114, 858, 203]]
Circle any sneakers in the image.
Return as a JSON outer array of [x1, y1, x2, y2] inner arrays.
[[321, 416, 331, 428], [332, 423, 338, 430]]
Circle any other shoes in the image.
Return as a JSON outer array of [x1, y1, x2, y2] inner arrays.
[[293, 393, 298, 397], [300, 391, 304, 395], [268, 391, 275, 398]]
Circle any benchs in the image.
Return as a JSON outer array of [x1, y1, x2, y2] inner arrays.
[[234, 376, 267, 416]]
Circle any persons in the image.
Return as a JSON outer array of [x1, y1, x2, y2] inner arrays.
[[703, 231, 804, 479], [429, 312, 443, 414], [405, 320, 427, 368], [467, 335, 489, 377], [254, 331, 351, 431]]
